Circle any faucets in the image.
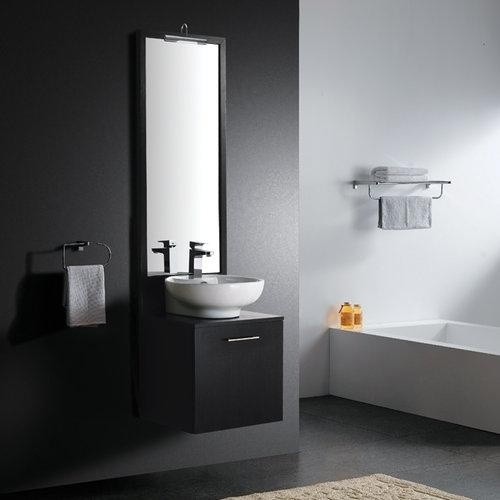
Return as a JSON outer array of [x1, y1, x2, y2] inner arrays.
[[152, 239, 175, 273], [189, 240, 212, 274]]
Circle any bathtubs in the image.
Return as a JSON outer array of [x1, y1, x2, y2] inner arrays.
[[329, 318, 500, 434]]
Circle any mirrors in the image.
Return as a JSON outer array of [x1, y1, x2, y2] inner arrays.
[[132, 28, 229, 282]]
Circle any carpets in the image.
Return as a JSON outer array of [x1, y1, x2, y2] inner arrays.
[[217, 473, 473, 499]]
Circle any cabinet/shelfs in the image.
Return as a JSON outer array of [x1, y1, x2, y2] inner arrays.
[[146, 309, 286, 435]]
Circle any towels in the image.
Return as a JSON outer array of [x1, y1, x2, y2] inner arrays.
[[370, 163, 435, 230], [62, 264, 107, 328]]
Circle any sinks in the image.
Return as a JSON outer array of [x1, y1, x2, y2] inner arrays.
[[163, 275, 262, 320]]
